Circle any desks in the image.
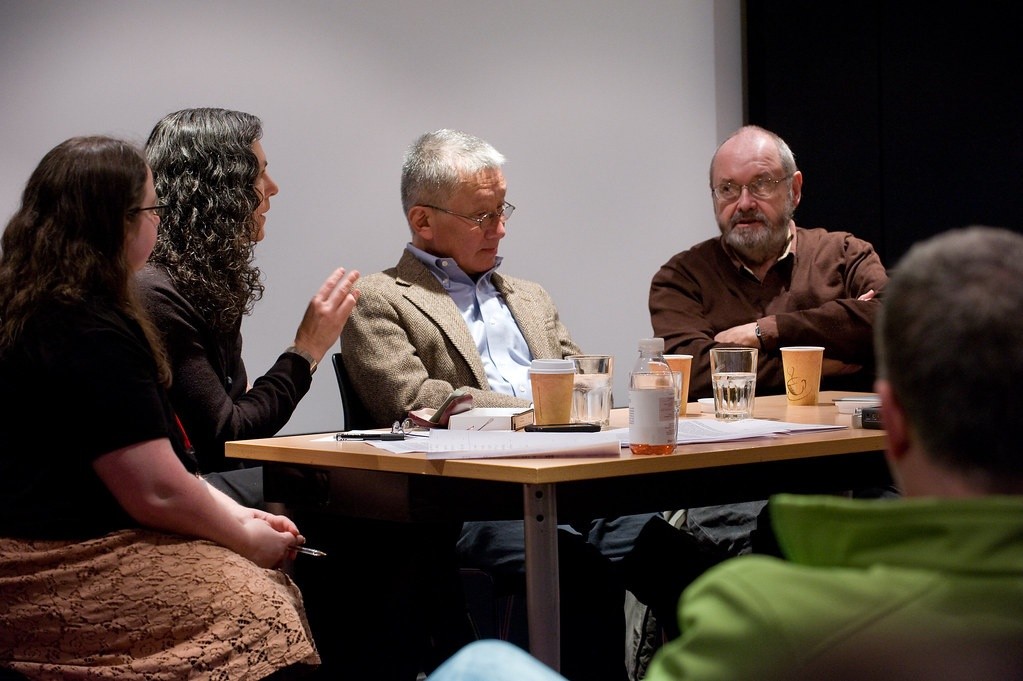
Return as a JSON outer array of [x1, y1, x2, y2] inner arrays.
[[223, 393, 894, 670]]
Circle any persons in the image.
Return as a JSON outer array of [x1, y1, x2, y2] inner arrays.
[[649, 124, 889, 557], [143, 107, 360, 519], [424, 227, 1023, 681], [339, 129, 719, 681], [0, 132, 321, 681]]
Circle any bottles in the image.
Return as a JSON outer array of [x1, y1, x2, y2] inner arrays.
[[628, 336, 678, 455]]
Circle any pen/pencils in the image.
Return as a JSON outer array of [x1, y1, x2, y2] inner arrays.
[[289, 544, 327, 557], [336, 433, 404, 440]]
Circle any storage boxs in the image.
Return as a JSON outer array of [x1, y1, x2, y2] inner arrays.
[[450, 407, 534, 430]]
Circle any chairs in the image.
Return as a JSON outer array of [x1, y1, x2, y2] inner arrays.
[[332, 354, 376, 432]]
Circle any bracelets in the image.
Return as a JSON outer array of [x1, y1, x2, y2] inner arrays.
[[286, 345, 318, 375]]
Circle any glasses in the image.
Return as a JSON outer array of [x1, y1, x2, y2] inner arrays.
[[126, 200, 171, 219], [391, 418, 431, 438], [416, 200, 515, 230], [712, 174, 793, 201]]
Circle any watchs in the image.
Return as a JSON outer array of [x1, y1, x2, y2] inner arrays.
[[755, 324, 763, 345]]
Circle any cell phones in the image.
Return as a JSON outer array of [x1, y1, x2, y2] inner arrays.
[[525, 423, 602, 432]]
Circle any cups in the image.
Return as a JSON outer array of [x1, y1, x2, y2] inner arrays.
[[780, 346, 825, 406], [529, 359, 576, 427], [709, 347, 759, 422], [564, 354, 614, 431], [648, 355, 694, 418]]
